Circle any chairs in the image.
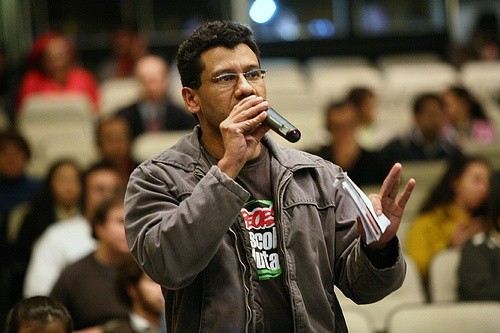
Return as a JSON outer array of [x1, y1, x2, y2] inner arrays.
[[343, 303, 377, 333], [332, 251, 423, 333], [383, 296, 500, 333], [428, 249, 460, 301], [24, 60, 500, 246]]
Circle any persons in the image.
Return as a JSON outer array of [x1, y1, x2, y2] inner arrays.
[[0, 28, 195, 333], [302, 85, 500, 302], [123, 20, 416, 333]]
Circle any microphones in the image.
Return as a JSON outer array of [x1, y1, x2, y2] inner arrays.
[[254, 99, 301, 143]]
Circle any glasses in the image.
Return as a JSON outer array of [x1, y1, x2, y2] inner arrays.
[[188, 69, 268, 87]]
